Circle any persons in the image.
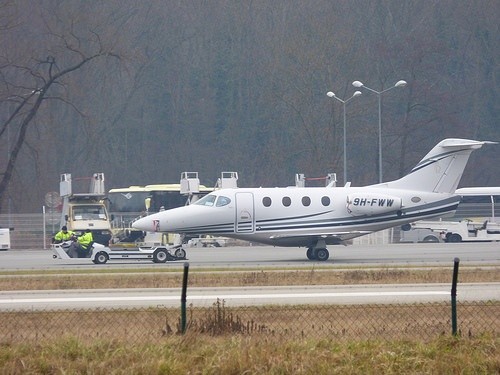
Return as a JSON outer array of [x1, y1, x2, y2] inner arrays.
[[68, 229, 93, 258], [54, 226, 73, 242]]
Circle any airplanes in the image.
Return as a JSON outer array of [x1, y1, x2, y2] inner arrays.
[[130, 137, 498, 261]]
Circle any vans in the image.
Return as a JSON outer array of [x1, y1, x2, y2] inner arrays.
[[451, 187, 500, 233]]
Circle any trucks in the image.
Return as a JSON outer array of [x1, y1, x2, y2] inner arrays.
[[57, 171, 113, 247]]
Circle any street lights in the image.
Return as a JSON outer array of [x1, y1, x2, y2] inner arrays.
[[326, 91, 363, 184], [353, 80, 407, 183]]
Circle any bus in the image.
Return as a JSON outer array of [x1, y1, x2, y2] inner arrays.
[[106, 181, 223, 229]]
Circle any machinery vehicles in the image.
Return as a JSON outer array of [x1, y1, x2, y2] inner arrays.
[[51, 238, 170, 265]]
